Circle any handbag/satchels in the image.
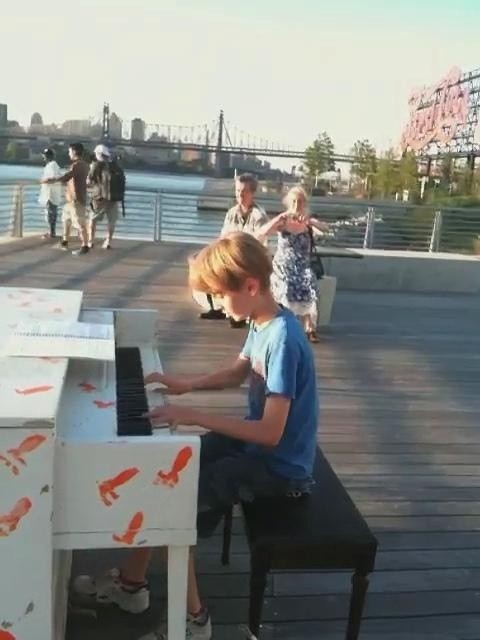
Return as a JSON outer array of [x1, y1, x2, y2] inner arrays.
[[309, 255, 324, 280]]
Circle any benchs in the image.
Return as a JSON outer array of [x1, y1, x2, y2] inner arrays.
[[323, 248, 480, 297], [222, 440, 380, 638]]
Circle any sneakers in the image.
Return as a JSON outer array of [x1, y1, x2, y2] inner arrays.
[[73, 568, 150, 614], [137, 608, 211, 640], [41, 234, 111, 255]]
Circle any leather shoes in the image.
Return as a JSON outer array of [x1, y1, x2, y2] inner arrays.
[[200, 310, 225, 319]]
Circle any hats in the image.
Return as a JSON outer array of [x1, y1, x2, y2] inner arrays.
[[94, 145, 111, 156]]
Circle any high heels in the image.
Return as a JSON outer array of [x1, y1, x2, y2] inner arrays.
[[306, 331, 320, 343]]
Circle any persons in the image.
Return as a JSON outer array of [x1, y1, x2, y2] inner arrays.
[[93, 230, 321, 639], [39, 149, 61, 239], [254, 188, 329, 344], [87, 144, 119, 249], [38, 142, 89, 256], [197, 172, 270, 328]]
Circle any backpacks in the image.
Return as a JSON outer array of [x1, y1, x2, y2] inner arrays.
[[93, 158, 125, 201]]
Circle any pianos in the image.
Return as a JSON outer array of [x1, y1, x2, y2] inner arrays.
[[2, 288, 201, 638]]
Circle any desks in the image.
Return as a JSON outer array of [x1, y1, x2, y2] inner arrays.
[[311, 242, 364, 278]]
